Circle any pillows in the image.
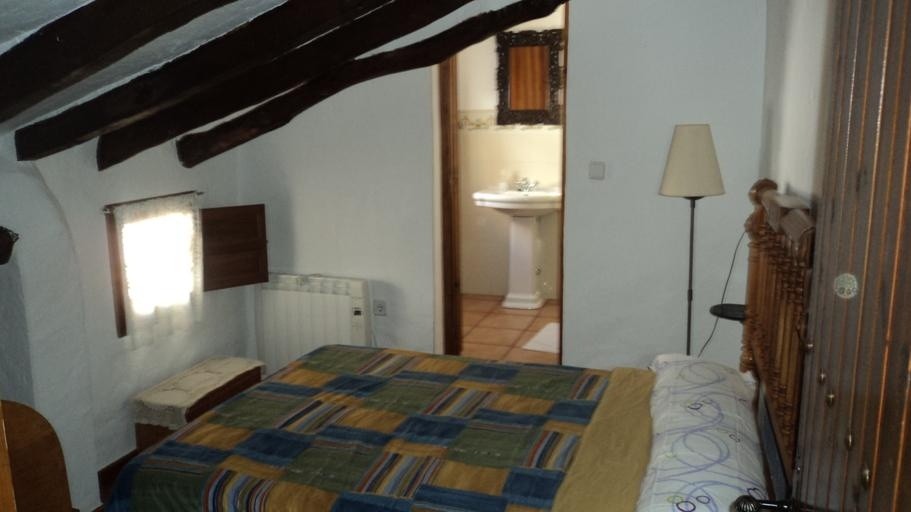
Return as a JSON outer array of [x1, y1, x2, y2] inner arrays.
[[636, 359, 774, 512]]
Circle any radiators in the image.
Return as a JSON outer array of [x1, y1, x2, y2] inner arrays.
[[258, 272, 370, 372]]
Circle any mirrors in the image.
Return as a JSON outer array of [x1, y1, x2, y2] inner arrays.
[[494, 28, 564, 125]]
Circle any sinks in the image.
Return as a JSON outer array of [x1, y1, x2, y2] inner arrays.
[[472, 186, 562, 217]]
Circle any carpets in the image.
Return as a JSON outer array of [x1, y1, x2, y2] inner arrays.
[[522, 320, 559, 355]]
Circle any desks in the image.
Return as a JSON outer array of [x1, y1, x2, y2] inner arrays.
[[132, 354, 260, 454]]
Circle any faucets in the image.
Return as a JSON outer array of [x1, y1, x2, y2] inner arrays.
[[515, 178, 538, 191]]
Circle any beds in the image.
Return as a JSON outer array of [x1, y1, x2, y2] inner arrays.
[[108, 179, 818, 512]]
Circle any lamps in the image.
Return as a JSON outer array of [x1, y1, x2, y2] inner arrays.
[[657, 122, 725, 356]]
